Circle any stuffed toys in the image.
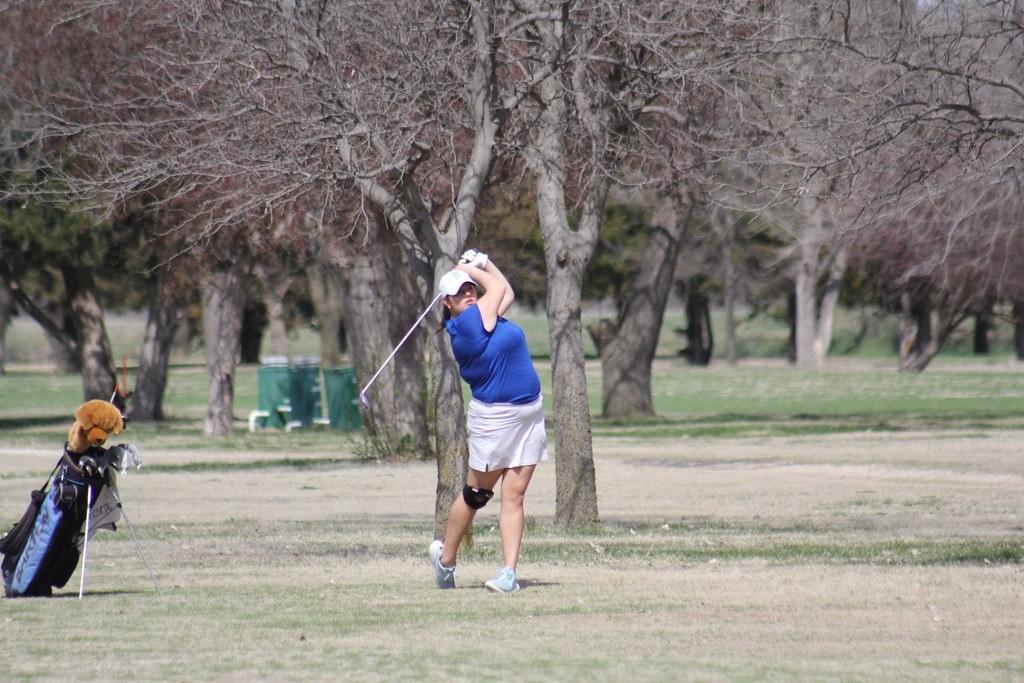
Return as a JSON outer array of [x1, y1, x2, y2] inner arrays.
[[67, 401, 123, 454]]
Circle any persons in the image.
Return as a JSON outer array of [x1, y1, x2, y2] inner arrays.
[[429, 250, 548, 595]]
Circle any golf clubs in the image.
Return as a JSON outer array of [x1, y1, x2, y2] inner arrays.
[[358, 246, 479, 412], [76, 441, 143, 487]]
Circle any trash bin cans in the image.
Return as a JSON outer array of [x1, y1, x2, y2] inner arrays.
[[248, 355, 361, 433]]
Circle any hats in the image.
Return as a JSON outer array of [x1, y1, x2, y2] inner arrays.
[[438, 270, 477, 300]]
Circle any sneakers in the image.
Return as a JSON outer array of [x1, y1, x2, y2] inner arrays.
[[428, 540, 456, 589], [484, 566, 519, 594]]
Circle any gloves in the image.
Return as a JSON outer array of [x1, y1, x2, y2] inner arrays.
[[458, 249, 488, 269]]
